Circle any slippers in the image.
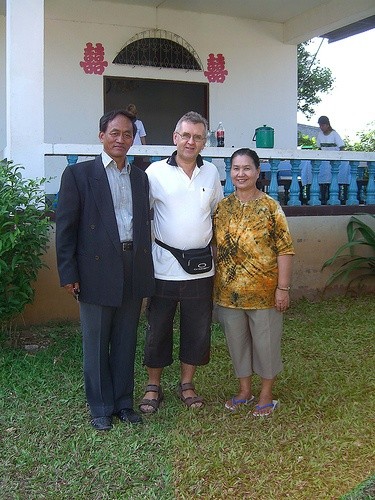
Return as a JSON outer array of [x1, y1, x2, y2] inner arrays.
[[224, 395, 255, 410], [253, 400, 278, 416]]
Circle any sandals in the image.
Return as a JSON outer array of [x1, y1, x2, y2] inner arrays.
[[138, 385, 163, 414], [177, 382, 205, 410]]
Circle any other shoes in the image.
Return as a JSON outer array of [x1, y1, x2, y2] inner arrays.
[[91, 416, 112, 430], [113, 409, 143, 424]]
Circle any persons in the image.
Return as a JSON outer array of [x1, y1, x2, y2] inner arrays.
[[55, 109, 151, 433], [214, 148, 295, 417], [140, 111, 223, 413], [127, 104, 148, 170], [314, 116, 346, 151]]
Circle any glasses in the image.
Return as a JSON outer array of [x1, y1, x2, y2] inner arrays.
[[176, 131, 205, 142]]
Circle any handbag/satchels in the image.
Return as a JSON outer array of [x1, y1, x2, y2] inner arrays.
[[154, 238, 213, 274]]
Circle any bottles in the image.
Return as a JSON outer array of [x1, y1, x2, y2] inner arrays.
[[216, 121, 225, 147], [206, 130, 216, 147]]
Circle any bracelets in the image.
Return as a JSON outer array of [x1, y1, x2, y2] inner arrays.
[[276, 285, 291, 291]]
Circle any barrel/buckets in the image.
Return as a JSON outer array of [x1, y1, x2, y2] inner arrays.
[[253, 125, 274, 148]]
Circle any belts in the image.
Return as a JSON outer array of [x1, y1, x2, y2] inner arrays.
[[120, 242, 133, 252]]
